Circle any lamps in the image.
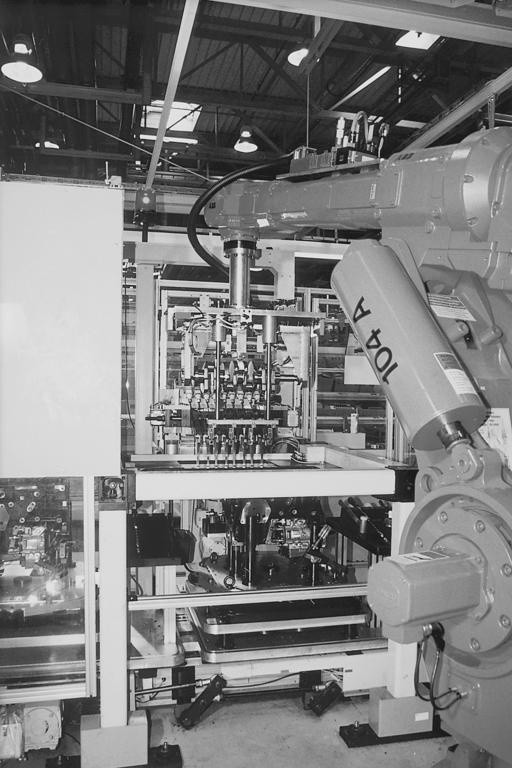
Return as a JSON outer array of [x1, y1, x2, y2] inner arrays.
[[234, 138, 258, 153], [35, 137, 60, 150], [240, 125, 253, 138], [287, 48, 321, 66], [0, 60, 44, 83], [396, 30, 442, 51], [13, 40, 32, 56]]
